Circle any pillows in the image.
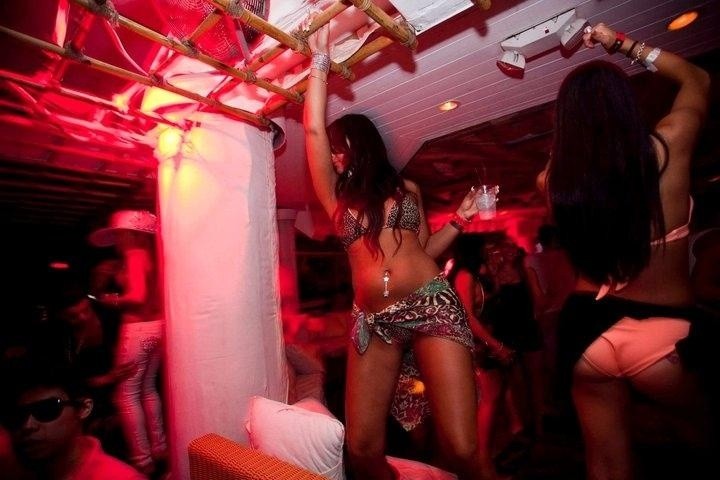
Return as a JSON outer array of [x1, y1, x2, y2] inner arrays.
[[247, 392, 348, 480], [243, 396, 336, 451]]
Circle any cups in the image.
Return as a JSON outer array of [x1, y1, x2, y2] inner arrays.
[[470, 184, 496, 221]]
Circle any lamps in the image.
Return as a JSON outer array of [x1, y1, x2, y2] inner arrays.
[[495, 5, 592, 80]]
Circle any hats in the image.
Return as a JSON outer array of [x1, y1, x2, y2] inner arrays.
[[88, 209, 156, 248]]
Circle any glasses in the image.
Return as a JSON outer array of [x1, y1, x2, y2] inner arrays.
[[8, 396, 80, 422]]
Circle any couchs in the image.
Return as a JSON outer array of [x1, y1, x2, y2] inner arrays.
[[185, 432, 460, 479]]
